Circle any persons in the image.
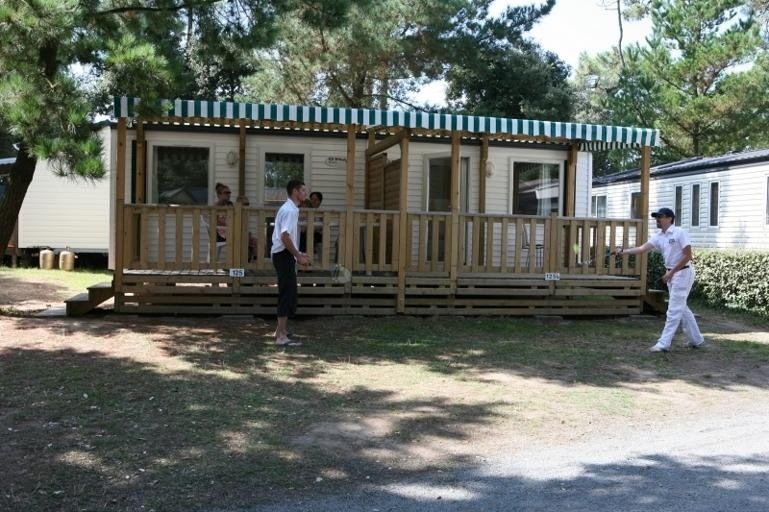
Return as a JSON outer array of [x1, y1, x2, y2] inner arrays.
[[209, 182, 258, 264], [609, 205, 709, 354], [270, 179, 315, 346], [294, 190, 325, 255]]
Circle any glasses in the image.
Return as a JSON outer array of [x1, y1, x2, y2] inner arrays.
[[221, 191, 231, 195]]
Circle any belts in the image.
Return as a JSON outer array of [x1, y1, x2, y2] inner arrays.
[[668, 265, 689, 272]]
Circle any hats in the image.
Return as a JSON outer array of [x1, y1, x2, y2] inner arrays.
[[651, 208, 676, 218]]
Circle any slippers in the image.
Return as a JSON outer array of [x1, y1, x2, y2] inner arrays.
[[276, 341, 303, 347], [273, 333, 301, 340]]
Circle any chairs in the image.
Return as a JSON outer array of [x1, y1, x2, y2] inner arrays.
[[521, 223, 544, 267], [200, 214, 229, 266]]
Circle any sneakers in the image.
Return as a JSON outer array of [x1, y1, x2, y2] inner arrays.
[[682, 341, 706, 348], [649, 346, 670, 352]]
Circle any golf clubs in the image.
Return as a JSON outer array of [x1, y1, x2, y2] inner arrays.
[[584, 249, 624, 264]]
